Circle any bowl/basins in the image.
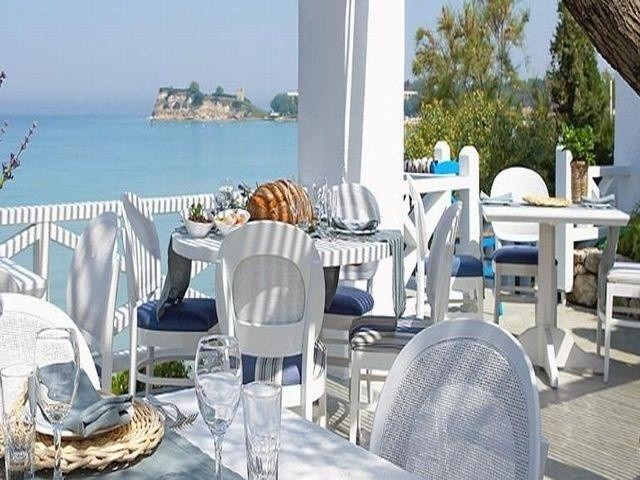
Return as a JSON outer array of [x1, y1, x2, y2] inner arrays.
[[181, 210, 214, 239], [214, 209, 251, 238]]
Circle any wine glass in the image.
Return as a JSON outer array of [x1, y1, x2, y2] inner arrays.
[[196, 334, 244, 480], [34, 327, 81, 480], [287, 179, 340, 238]]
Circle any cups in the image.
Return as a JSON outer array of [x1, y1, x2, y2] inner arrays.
[[1, 364, 34, 480], [242, 381, 282, 480]]
